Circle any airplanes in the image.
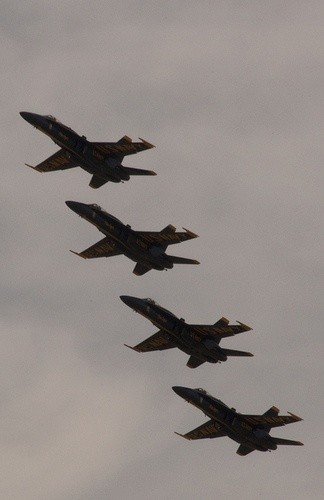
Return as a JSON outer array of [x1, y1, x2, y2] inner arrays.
[[65, 200, 200, 275], [119, 295, 255, 368], [170, 386, 304, 456], [18, 111, 157, 189]]
